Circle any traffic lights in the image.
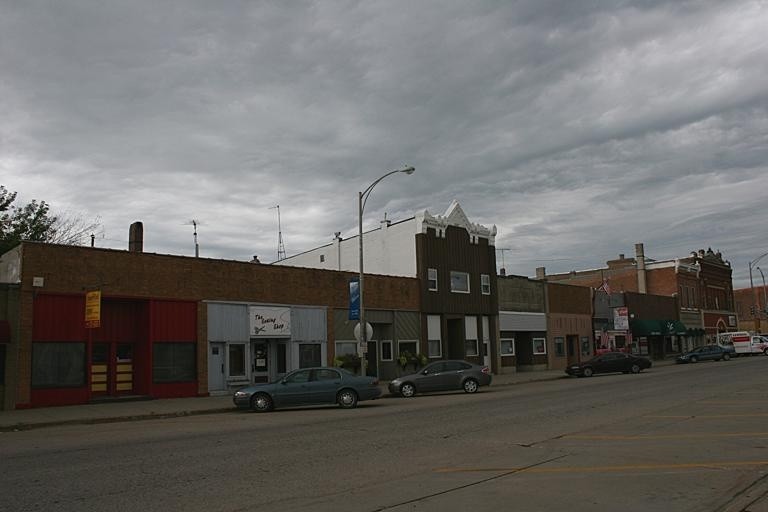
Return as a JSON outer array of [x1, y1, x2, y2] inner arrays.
[[750, 306, 754, 314]]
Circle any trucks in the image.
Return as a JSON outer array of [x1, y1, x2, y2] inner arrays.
[[731, 335, 768, 356]]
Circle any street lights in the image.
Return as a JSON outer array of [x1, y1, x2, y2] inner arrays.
[[757, 267, 766, 311], [354, 164, 417, 377]]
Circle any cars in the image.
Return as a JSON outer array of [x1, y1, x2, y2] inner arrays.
[[235, 364, 382, 410], [676, 343, 736, 365], [388, 357, 493, 396], [563, 351, 651, 378]]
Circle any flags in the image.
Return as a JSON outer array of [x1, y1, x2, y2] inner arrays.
[[601, 326, 608, 349], [627, 328, 633, 344], [603, 282, 614, 297]]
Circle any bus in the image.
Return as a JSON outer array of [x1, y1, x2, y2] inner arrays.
[[718, 329, 760, 349]]
